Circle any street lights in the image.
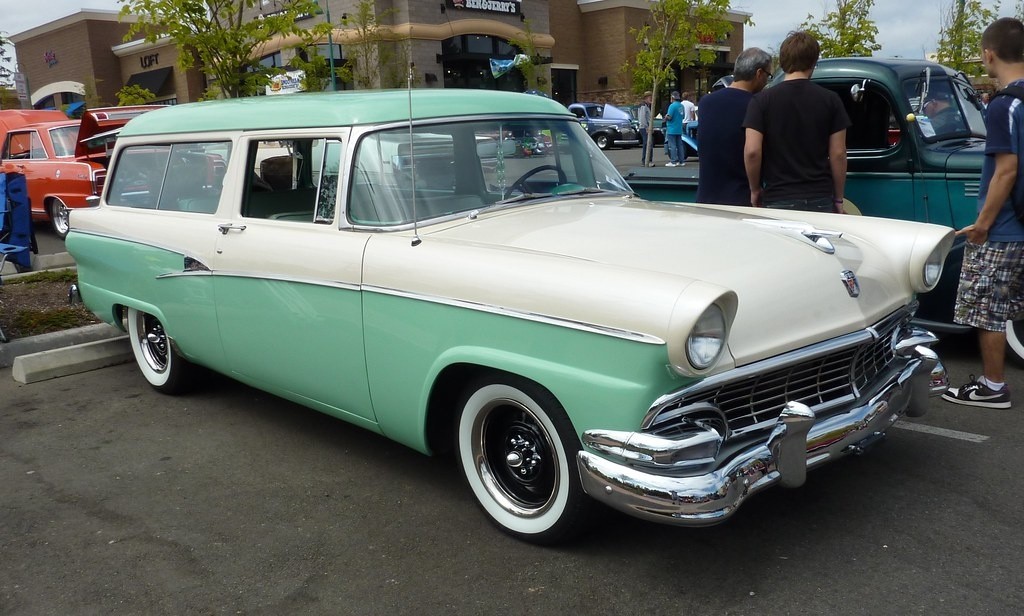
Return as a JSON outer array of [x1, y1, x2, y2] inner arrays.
[[312, 0, 336, 91]]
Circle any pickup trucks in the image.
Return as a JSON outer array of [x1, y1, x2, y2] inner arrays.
[[530, 102, 640, 155], [516, 56, 1024, 368], [614, 104, 667, 148]]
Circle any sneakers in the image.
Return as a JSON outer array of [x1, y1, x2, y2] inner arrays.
[[677, 162, 685, 167], [665, 162, 676, 167], [940, 374, 1012, 409]]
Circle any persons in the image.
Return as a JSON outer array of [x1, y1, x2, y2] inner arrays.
[[665, 91, 695, 167], [942, 17, 1024, 409], [919, 91, 966, 135], [979, 93, 989, 109], [638, 91, 655, 167], [697, 32, 852, 214]]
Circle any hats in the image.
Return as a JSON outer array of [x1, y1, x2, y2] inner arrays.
[[642, 91, 652, 100], [671, 91, 681, 99], [913, 94, 948, 112]]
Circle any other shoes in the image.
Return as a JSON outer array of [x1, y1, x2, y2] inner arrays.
[[649, 162, 655, 167], [641, 163, 645, 167]]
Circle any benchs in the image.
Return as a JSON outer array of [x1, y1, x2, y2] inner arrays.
[[180, 187, 318, 216]]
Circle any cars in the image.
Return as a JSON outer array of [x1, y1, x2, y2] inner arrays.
[[67, 84, 954, 549], [0, 104, 226, 241], [204, 131, 514, 190]]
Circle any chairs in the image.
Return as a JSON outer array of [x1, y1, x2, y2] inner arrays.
[[415, 156, 459, 216]]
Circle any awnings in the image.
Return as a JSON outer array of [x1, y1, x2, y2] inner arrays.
[[663, 58, 735, 69]]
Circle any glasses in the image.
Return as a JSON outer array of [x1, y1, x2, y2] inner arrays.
[[922, 99, 936, 110], [761, 68, 774, 80], [813, 64, 818, 69]]
